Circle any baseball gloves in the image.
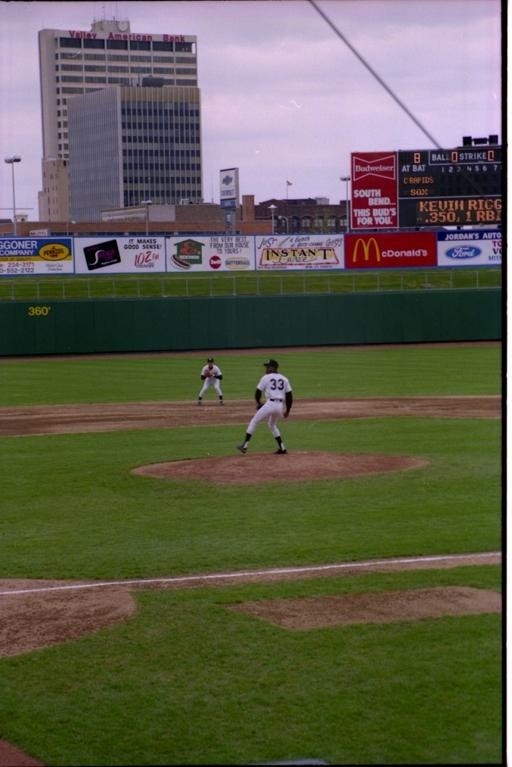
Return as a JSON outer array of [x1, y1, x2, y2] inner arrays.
[[256, 403, 264, 410], [204, 369, 212, 377]]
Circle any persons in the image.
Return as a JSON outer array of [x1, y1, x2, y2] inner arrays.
[[198, 357, 224, 405], [235, 359, 292, 454]]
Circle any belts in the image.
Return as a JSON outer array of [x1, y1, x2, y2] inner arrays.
[[270, 399, 283, 402]]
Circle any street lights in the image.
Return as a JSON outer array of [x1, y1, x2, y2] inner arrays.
[[339, 176, 351, 233], [66, 216, 77, 235], [141, 200, 153, 236], [268, 179, 294, 234], [4, 153, 22, 236]]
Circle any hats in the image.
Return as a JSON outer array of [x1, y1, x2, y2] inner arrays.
[[261, 357, 279, 370], [206, 357, 215, 361]]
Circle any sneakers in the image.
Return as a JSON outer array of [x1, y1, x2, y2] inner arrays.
[[198, 399, 224, 407], [273, 448, 289, 455], [235, 443, 247, 454]]
